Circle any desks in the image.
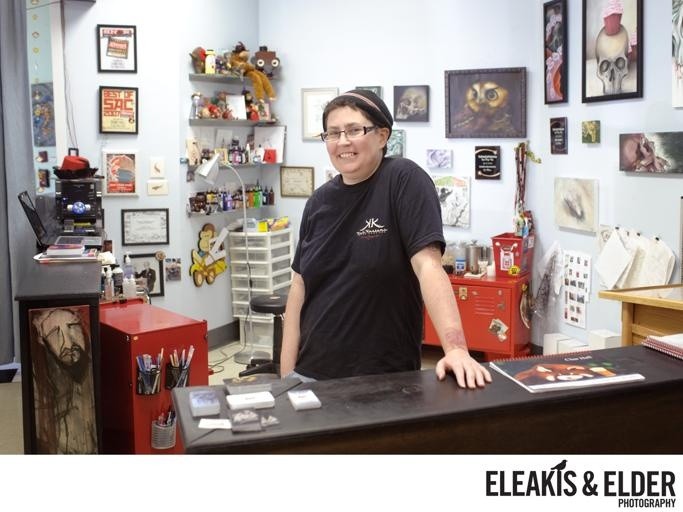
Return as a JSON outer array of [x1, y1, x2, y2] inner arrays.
[[168, 345, 681, 454], [11, 259, 104, 454]]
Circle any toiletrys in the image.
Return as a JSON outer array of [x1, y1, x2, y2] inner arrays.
[[100, 265, 114, 302], [122, 251, 135, 279]]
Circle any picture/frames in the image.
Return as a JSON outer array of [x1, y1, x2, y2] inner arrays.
[[541, 0, 568, 106], [299, 85, 341, 144], [443, 67, 527, 140], [252, 124, 286, 166], [98, 85, 138, 136], [95, 22, 139, 74], [279, 166, 316, 200], [120, 207, 170, 246], [580, 0, 644, 104], [122, 252, 165, 297], [98, 146, 142, 197]]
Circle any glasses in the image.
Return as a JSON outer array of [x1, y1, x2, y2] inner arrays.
[[319, 125, 374, 142]]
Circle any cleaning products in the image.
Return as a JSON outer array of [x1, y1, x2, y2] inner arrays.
[[112, 263, 125, 294]]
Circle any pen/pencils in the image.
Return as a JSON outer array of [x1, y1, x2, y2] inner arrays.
[[169, 345, 195, 388], [136, 347, 164, 394], [157, 411, 177, 426]]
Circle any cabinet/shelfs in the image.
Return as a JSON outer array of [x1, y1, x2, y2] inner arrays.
[[95, 297, 210, 454], [185, 72, 280, 217], [597, 284, 683, 347], [419, 271, 532, 360]]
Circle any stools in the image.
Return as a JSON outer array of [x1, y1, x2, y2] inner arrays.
[[237, 294, 289, 378]]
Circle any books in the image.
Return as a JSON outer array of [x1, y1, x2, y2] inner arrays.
[[489, 350, 646, 393], [641, 332, 683, 360]]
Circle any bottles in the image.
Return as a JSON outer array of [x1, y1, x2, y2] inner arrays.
[[466, 239, 484, 274], [256, 144, 265, 161], [228, 143, 251, 164], [194, 178, 274, 211], [122, 274, 136, 298], [455, 258, 467, 276]]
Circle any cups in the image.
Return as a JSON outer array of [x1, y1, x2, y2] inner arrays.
[[486, 260, 495, 278], [477, 255, 488, 271]]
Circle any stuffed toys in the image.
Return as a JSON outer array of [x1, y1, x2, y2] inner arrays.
[[188, 41, 280, 121]]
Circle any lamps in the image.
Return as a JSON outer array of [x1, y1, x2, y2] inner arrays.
[[195, 151, 271, 366]]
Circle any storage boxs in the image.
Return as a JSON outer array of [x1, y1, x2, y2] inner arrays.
[[226, 227, 297, 353]]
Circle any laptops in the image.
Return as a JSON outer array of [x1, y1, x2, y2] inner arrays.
[[18, 190, 102, 250]]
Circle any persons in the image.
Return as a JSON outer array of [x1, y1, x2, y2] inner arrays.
[[279, 89, 492, 389], [29, 310, 97, 455], [137, 262, 156, 293]]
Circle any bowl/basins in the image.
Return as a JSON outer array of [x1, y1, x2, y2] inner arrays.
[[51, 167, 98, 180]]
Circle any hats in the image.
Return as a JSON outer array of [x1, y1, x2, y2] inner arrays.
[[331, 89, 392, 139]]
